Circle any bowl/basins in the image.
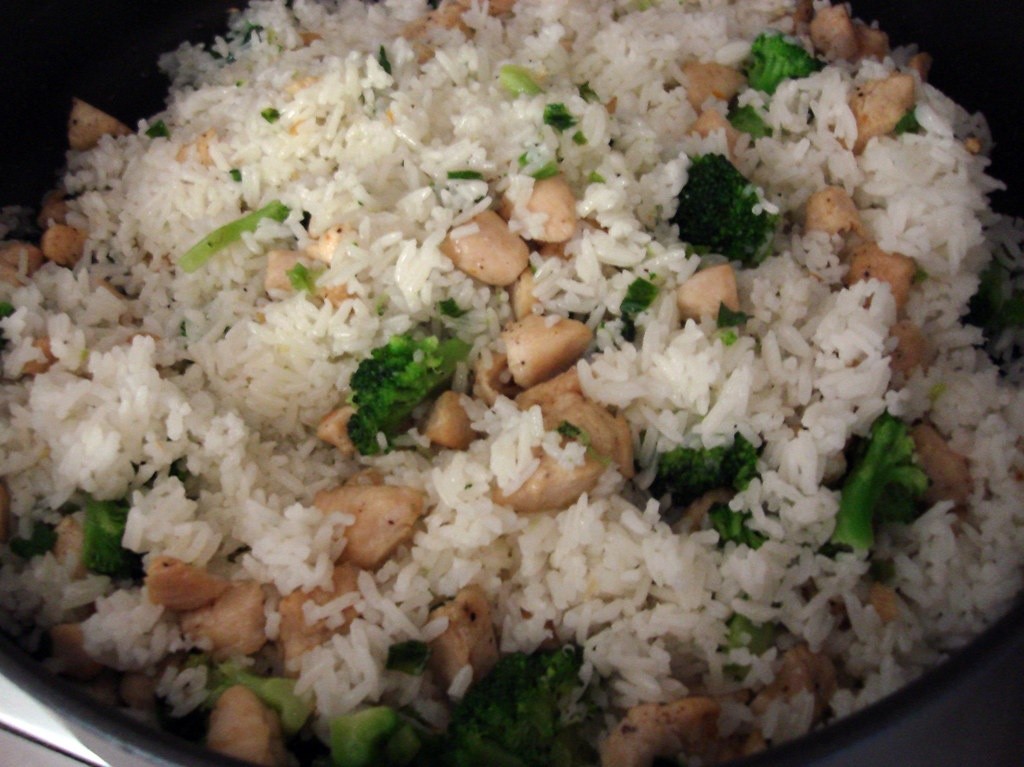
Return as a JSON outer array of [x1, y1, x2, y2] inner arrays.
[[0, 0, 1023, 766]]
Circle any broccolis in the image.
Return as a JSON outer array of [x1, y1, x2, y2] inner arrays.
[[10, 19, 1023, 767]]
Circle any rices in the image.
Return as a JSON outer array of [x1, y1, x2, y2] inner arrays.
[[0, 0, 1024, 767]]
